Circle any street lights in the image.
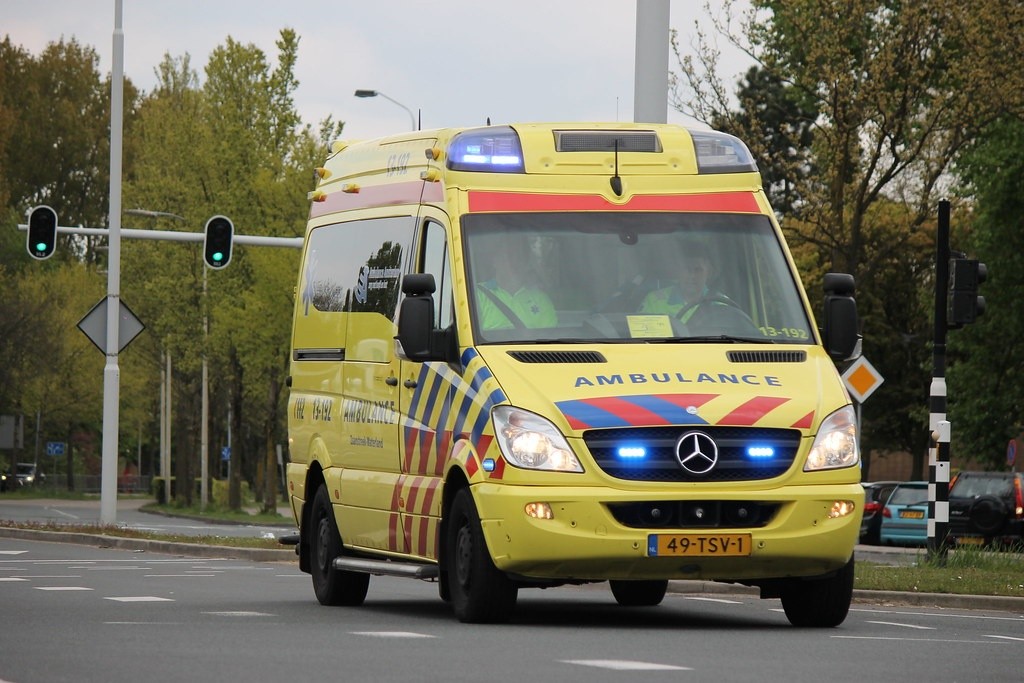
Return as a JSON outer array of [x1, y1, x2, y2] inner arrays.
[[355, 89, 417, 131]]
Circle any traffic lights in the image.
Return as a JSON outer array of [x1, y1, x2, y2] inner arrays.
[[26, 205, 59, 261], [947, 258, 990, 323], [202, 215, 235, 271]]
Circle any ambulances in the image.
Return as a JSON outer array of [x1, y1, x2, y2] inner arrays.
[[285, 119, 859, 630]]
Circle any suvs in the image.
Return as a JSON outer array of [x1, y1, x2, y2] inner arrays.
[[945, 469, 1023, 550]]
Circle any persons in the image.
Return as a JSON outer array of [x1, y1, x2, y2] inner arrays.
[[635, 247, 730, 325], [474, 232, 559, 330]]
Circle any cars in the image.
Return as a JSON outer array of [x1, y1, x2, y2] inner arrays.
[[879, 481, 933, 548], [0, 463, 49, 494], [860, 480, 896, 546]]
